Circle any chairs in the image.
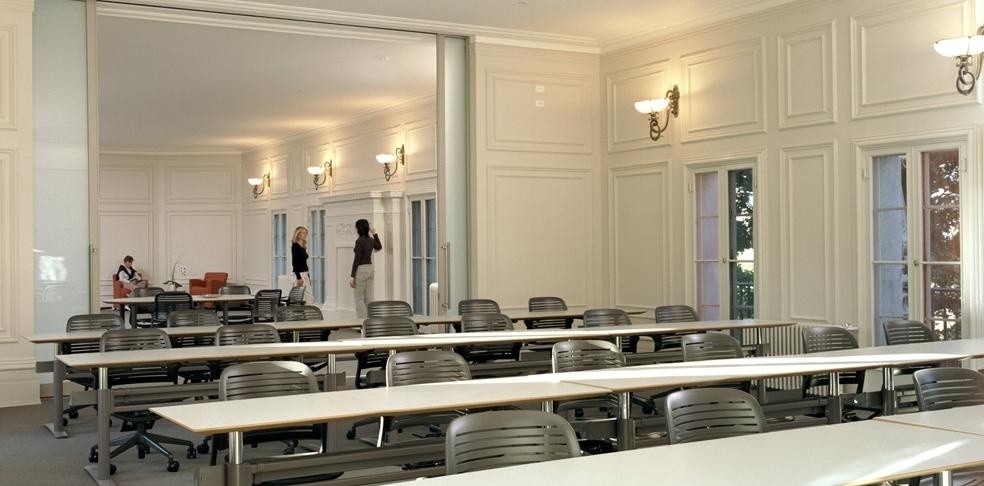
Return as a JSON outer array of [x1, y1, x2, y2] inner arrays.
[[218, 286, 253, 322], [133, 287, 164, 314], [444, 410, 581, 476], [344, 315, 462, 440], [664, 387, 769, 445], [914, 367, 984, 413], [62, 314, 124, 427], [366, 301, 414, 318], [461, 314, 515, 353], [167, 309, 220, 381], [376, 348, 474, 447], [456, 300, 501, 331], [150, 292, 193, 328], [274, 304, 328, 371], [252, 289, 282, 323], [219, 359, 324, 459], [882, 319, 939, 372], [642, 305, 702, 414], [681, 333, 751, 394], [285, 286, 306, 306], [525, 297, 572, 328], [575, 309, 659, 416], [198, 325, 299, 461], [549, 339, 628, 422], [89, 328, 198, 474], [800, 327, 865, 421]]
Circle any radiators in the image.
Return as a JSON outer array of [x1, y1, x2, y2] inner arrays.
[[742, 320, 858, 396]]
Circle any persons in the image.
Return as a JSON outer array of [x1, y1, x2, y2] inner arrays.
[[291, 226, 318, 318], [350, 219, 382, 333], [117, 255, 147, 311]]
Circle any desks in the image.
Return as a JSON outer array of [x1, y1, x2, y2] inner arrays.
[[50, 327, 610, 485], [148, 374, 613, 485], [567, 315, 797, 420], [872, 393, 984, 486], [19, 309, 647, 440], [101, 293, 289, 328], [395, 418, 984, 486], [528, 334, 984, 453]]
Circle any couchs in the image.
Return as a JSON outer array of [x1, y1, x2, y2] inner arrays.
[[112, 273, 140, 309], [189, 271, 228, 309]]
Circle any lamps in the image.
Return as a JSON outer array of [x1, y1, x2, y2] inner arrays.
[[247, 172, 270, 199], [375, 144, 404, 182], [932, 25, 984, 96], [306, 160, 333, 191], [634, 85, 680, 141]]
